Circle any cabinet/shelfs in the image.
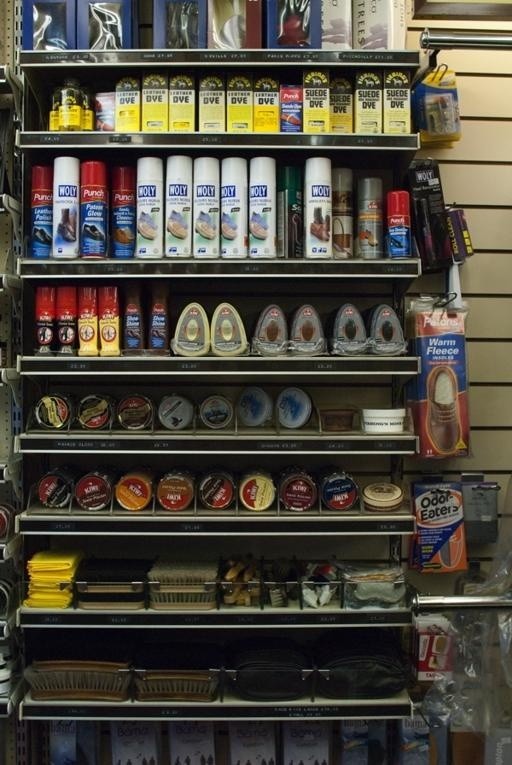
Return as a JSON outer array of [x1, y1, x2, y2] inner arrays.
[[1, 1, 421, 764]]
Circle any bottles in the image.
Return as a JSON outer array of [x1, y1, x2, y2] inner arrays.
[[48, 76, 94, 131], [35, 284, 169, 361], [30, 155, 415, 260]]
